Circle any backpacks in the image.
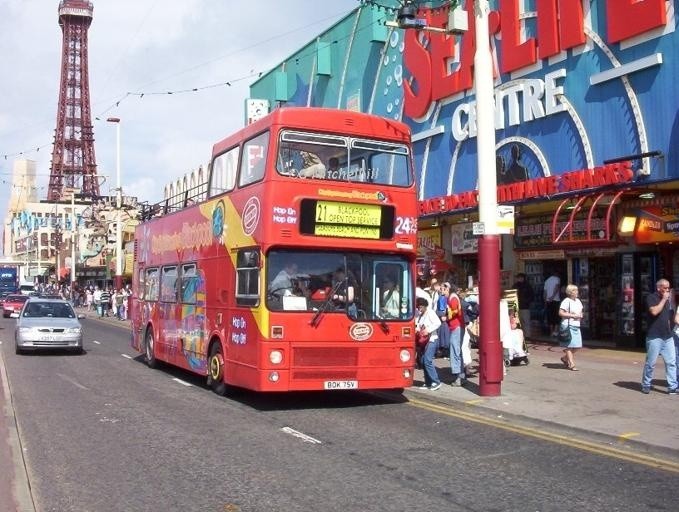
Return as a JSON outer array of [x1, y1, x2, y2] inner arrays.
[[459, 299, 479, 325]]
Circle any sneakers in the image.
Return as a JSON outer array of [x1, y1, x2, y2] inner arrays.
[[418, 372, 471, 391], [668, 388, 679, 395], [640, 386, 650, 393]]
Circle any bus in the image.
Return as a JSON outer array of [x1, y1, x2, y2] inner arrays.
[[133, 103, 422, 400]]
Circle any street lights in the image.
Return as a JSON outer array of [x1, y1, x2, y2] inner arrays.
[[64, 189, 76, 291], [106, 117, 125, 289]]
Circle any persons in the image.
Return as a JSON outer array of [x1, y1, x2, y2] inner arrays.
[[638, 278, 679, 396], [674, 303, 679, 339], [31, 279, 133, 322], [557, 284, 586, 371], [415, 268, 564, 393], [324, 155, 348, 179], [150, 204, 162, 219], [270, 261, 399, 315], [186, 198, 195, 207]]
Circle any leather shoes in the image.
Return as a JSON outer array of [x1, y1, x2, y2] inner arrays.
[[567, 364, 578, 371]]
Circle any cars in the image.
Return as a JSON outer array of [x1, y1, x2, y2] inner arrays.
[[0, 265, 87, 356]]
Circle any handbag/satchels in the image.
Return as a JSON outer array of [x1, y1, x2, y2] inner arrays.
[[415, 325, 430, 348], [557, 327, 572, 341]]
